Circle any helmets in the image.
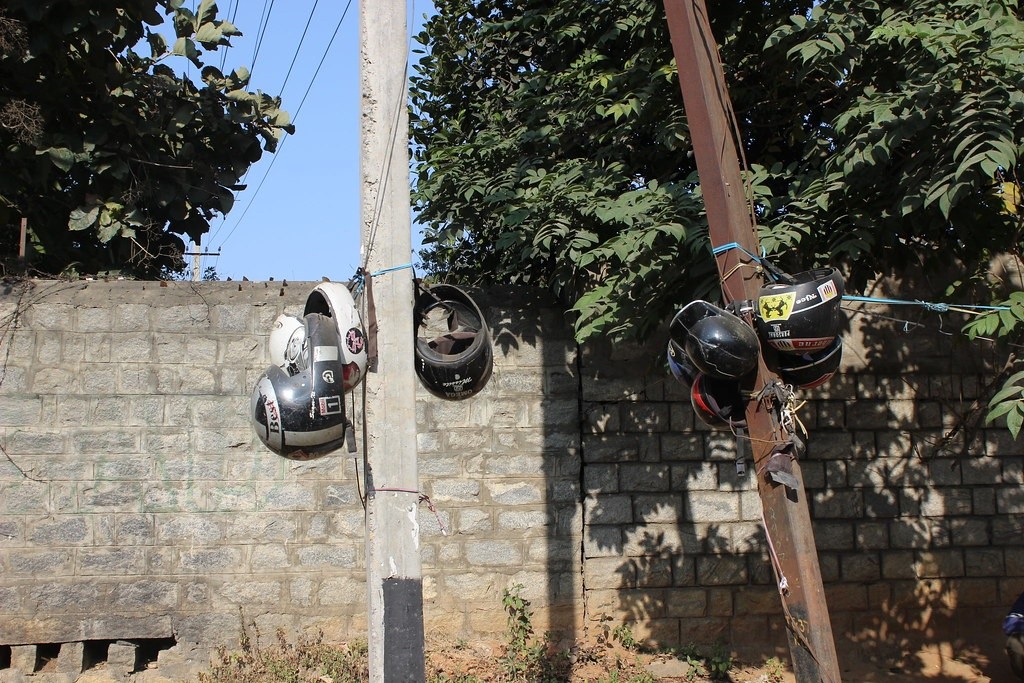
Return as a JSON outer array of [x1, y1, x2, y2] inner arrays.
[[667, 300, 758, 476], [413, 284, 493, 400], [754, 267, 845, 353], [761, 335, 842, 389], [268, 282, 368, 395], [251, 313, 346, 461]]
